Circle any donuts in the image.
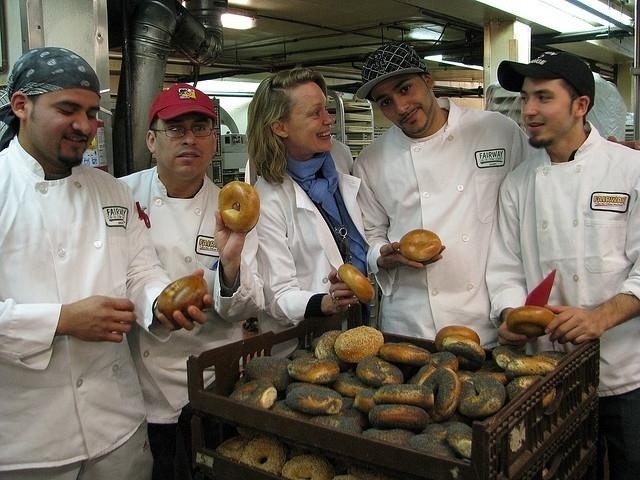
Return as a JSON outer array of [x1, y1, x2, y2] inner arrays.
[[227, 326, 561, 462]]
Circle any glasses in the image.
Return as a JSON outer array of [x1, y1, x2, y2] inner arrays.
[[151, 124, 215, 137]]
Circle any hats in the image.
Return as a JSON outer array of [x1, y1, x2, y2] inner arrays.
[[357, 43, 430, 99], [497, 51, 595, 109], [149, 83, 217, 130]]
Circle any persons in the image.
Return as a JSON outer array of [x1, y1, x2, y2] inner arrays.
[[113, 82, 269, 478], [349, 43, 532, 351], [251, 69, 378, 335], [1, 47, 214, 479], [485, 48, 638, 479]]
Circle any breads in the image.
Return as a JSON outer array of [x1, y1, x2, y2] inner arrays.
[[400, 230, 440, 263], [211, 426, 388, 480], [337, 263, 374, 302], [156, 276, 207, 324], [506, 306, 556, 339], [217, 181, 259, 234]]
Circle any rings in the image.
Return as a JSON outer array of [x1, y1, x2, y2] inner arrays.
[[331, 291, 335, 300]]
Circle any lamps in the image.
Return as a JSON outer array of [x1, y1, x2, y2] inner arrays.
[[475, 0, 637, 36]]
